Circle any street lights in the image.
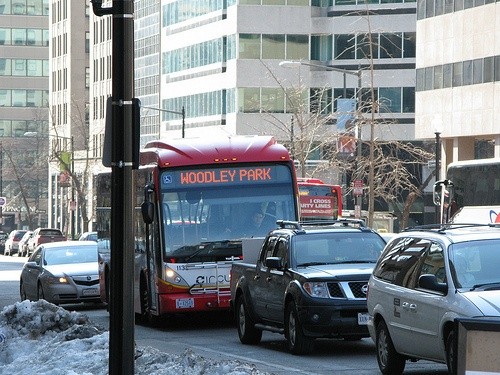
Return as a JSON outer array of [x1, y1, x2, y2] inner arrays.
[[23, 131, 75, 240], [278, 61, 363, 220]]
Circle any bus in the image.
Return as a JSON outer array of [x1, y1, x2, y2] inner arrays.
[[441, 157, 500, 228], [87, 133, 300, 325], [296, 177, 342, 226]]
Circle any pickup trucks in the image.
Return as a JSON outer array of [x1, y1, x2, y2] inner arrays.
[[228, 217, 391, 356]]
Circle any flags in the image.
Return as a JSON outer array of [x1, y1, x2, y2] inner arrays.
[[336, 99, 356, 153], [60, 152, 72, 187]]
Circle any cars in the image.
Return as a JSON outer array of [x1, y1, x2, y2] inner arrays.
[[78, 231, 98, 241], [19, 240, 102, 307], [0, 227, 68, 257]]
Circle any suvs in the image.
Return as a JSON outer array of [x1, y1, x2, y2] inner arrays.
[[363, 222, 500, 375]]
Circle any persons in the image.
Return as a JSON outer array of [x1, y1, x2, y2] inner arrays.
[[240, 210, 269, 237]]
